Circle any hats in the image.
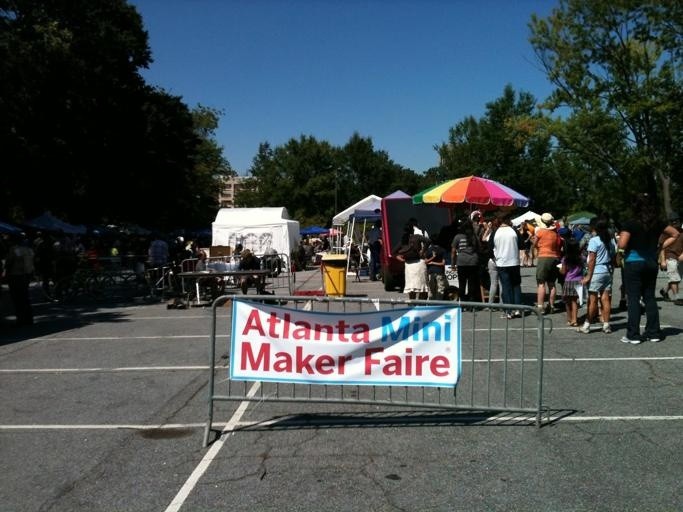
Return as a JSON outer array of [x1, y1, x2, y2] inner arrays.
[[534, 212, 556, 230]]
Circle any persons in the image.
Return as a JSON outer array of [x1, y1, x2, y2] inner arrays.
[[483, 211, 526, 318], [616, 187, 678, 345], [579, 211, 616, 334], [332, 210, 683, 345], [0, 208, 329, 327]]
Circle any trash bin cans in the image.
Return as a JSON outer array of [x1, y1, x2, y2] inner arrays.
[[320, 254, 347, 296]]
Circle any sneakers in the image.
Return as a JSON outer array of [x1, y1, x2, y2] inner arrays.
[[532, 307, 544, 314], [501, 313, 512, 318], [576, 325, 590, 333], [513, 310, 520, 316], [601, 326, 612, 332], [620, 336, 641, 345]]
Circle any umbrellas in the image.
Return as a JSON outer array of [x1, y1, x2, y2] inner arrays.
[[409, 173, 533, 223]]
